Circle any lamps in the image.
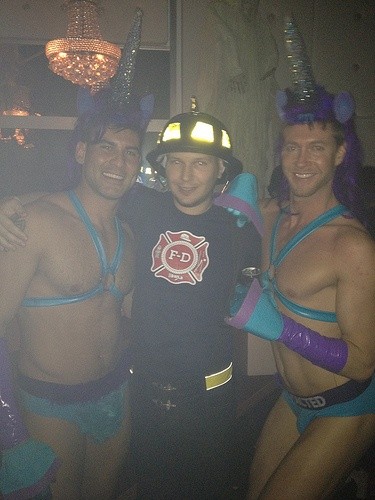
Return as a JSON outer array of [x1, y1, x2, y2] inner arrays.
[[45, 0, 121, 87]]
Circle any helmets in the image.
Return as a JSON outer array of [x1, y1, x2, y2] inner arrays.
[[147, 96, 243, 184]]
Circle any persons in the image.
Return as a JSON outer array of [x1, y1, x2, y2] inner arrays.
[[223, 93, 374, 500], [0, 113, 263, 499], [0, 89, 145, 500]]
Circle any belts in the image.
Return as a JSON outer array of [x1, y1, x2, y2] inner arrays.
[[146, 363, 233, 393]]
